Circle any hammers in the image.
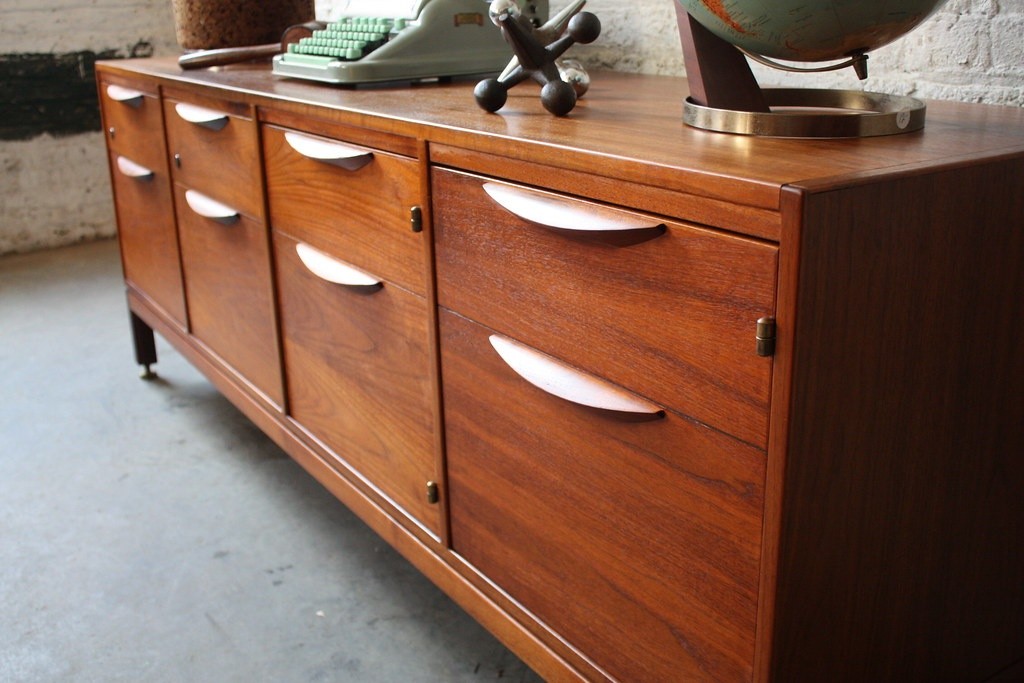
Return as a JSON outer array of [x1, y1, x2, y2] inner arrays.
[[179, 21, 326, 71]]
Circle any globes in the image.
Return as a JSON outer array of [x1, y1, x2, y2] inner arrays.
[[674, 0, 953, 138]]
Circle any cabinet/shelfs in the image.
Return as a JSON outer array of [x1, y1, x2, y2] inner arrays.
[[99, 79, 187, 331], [252, 103, 445, 544], [161, 82, 285, 412], [418, 136, 1024, 683]]
